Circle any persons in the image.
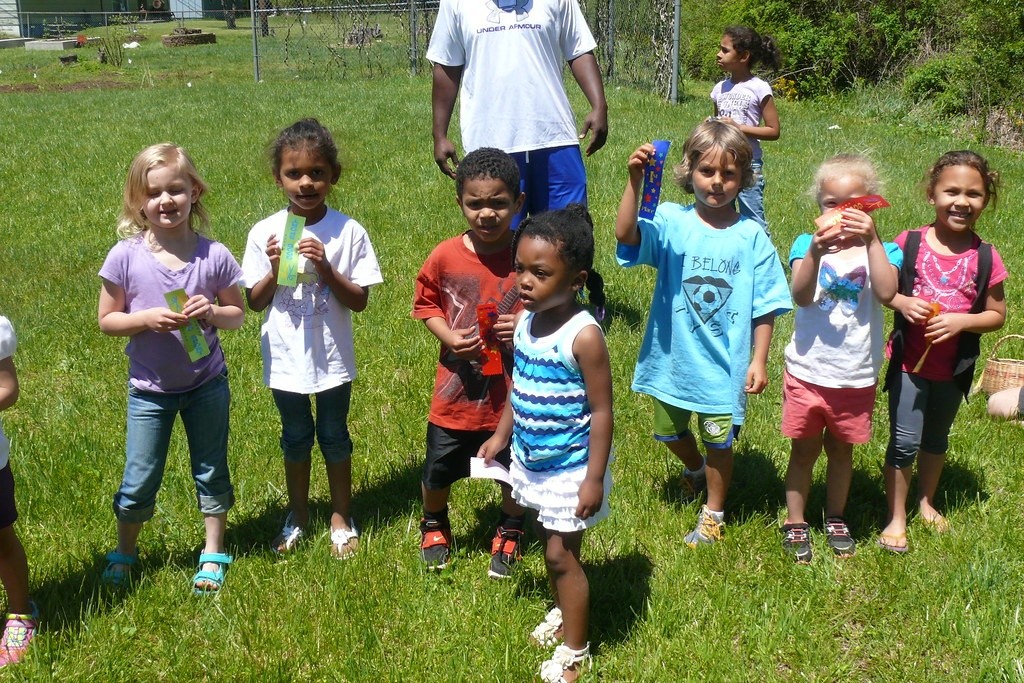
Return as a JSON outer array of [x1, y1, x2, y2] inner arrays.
[[476, 203, 613, 683], [97, 142, 245, 596], [709, 25, 780, 247], [880, 150, 1006, 552], [780, 154, 902, 562], [0, 312, 38, 670], [413, 149, 533, 578], [616, 121, 763, 520], [426, 0, 608, 328], [242, 121, 382, 560]]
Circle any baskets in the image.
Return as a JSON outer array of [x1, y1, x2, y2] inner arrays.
[[983, 336, 1024, 394]]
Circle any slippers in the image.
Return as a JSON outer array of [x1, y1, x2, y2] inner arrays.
[[917, 508, 948, 533], [878, 531, 908, 552]]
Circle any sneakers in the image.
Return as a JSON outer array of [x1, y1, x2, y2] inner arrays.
[[780, 519, 812, 566], [826, 518, 855, 557], [419, 513, 451, 569], [0, 612, 38, 668], [677, 474, 705, 501], [487, 525, 524, 578], [682, 510, 726, 548]]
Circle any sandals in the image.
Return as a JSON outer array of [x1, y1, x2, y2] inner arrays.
[[529, 609, 563, 649], [272, 511, 311, 555], [539, 641, 592, 683], [330, 517, 358, 559], [102, 547, 141, 587], [191, 548, 233, 596]]
